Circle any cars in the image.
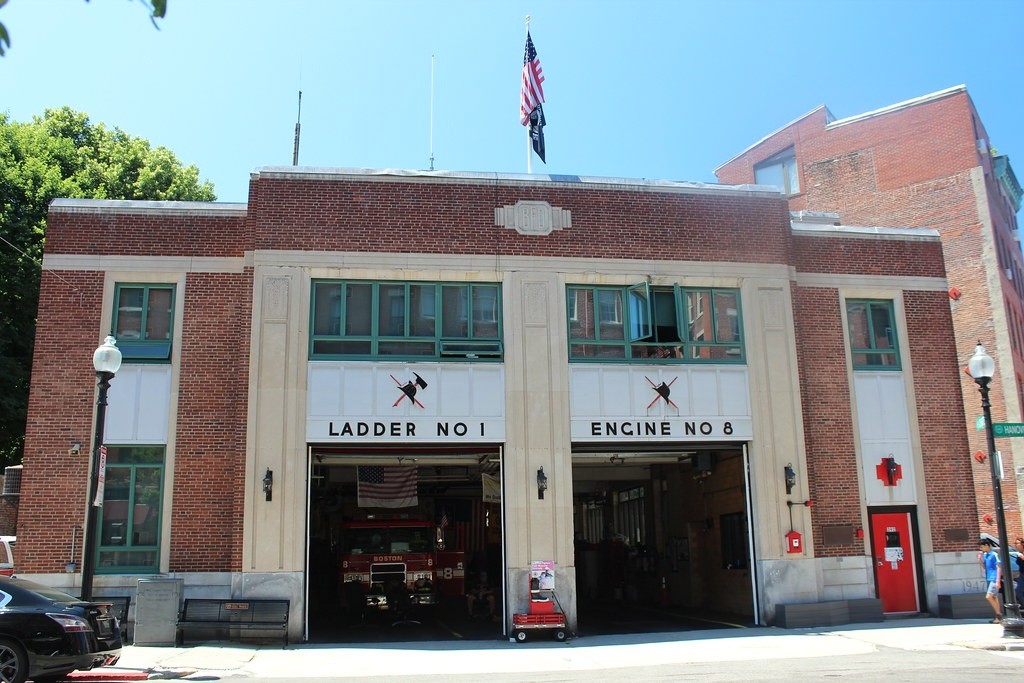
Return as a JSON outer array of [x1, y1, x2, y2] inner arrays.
[[0, 572, 124, 683], [980, 530, 1022, 591]]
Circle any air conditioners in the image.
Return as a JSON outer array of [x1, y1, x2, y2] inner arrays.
[[3, 465, 22, 496]]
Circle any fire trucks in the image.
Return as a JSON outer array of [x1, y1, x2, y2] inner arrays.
[[327, 493, 465, 630]]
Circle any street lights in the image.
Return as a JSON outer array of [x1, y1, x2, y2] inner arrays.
[[80, 332, 123, 603], [965, 338, 1024, 638]]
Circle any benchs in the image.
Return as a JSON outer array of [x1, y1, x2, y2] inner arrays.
[[174, 597, 291, 650], [75, 596, 131, 643]]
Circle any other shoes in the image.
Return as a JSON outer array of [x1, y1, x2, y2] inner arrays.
[[991, 615, 1002, 623]]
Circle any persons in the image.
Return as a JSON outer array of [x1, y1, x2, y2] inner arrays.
[[1015, 538, 1024, 618], [976, 538, 1002, 624]]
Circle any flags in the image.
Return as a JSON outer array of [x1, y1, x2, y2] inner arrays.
[[520, 15, 546, 164], [356, 463, 418, 507], [441, 505, 448, 529]]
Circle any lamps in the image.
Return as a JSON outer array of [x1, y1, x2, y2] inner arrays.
[[784, 462, 796, 494], [886, 454, 898, 485], [261, 467, 273, 501], [536, 466, 548, 500]]
